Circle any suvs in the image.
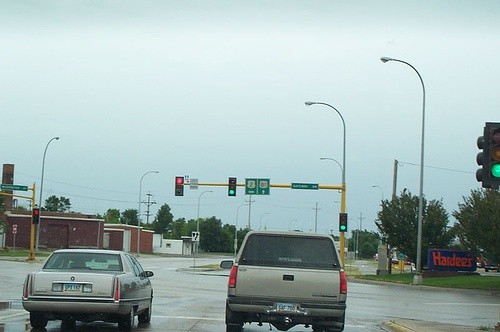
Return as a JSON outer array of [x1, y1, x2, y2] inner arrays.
[[220, 231, 347, 332]]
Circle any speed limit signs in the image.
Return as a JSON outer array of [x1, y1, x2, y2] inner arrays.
[[244, 177, 271, 196]]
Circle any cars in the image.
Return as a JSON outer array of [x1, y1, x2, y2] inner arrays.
[[21, 249, 155, 328], [477, 255, 500, 272]]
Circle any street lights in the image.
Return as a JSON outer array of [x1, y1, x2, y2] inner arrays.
[[304, 101, 347, 185], [235, 204, 249, 239], [260, 212, 271, 230], [381, 57, 425, 284], [372, 184, 385, 199], [34, 136, 60, 250], [320, 157, 342, 170], [137, 170, 160, 255], [197, 190, 214, 230]]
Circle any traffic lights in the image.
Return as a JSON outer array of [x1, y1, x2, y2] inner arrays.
[[174, 176, 184, 196], [475, 121, 500, 189], [228, 177, 236, 196], [32, 209, 39, 224]]
